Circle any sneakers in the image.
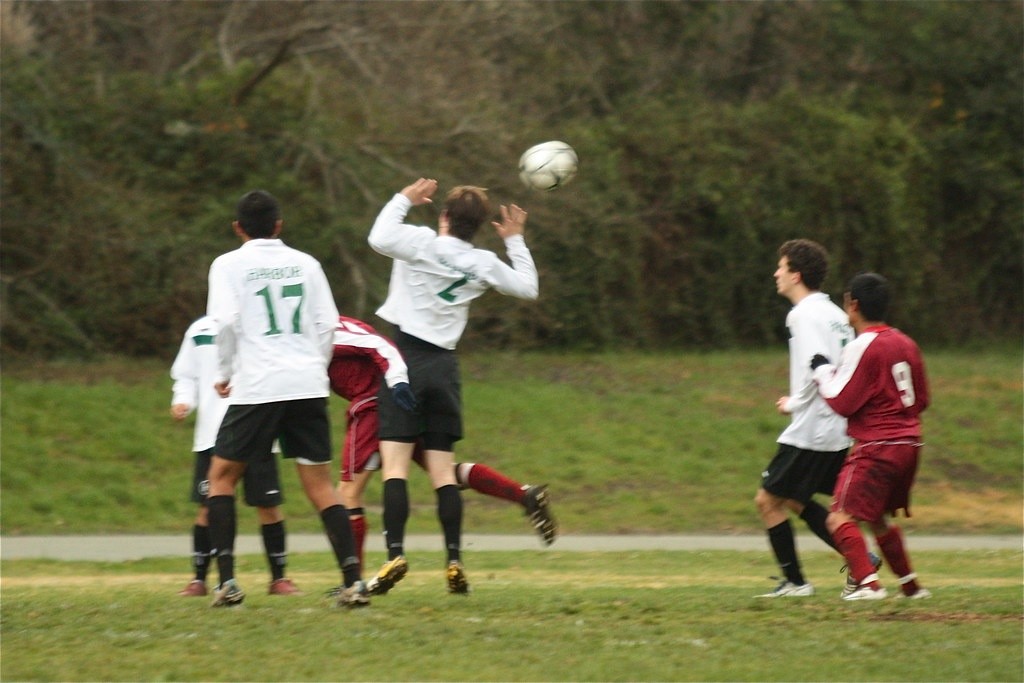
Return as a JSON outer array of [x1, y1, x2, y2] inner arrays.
[[210, 578, 246, 608], [335, 580, 370, 609], [366, 555, 408, 596], [839, 552, 883, 597], [448, 562, 468, 593], [752, 576, 815, 598], [522, 483, 558, 544], [269, 578, 301, 596], [179, 580, 207, 598], [894, 589, 933, 602], [844, 585, 890, 600]]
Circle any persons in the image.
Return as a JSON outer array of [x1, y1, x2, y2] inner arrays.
[[364, 176, 541, 595], [170, 316, 303, 597], [207, 191, 369, 608], [753, 239, 881, 599], [326, 316, 556, 596], [810, 274, 932, 601]]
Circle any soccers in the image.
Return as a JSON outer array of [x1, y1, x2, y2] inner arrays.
[[517, 139, 582, 194]]
